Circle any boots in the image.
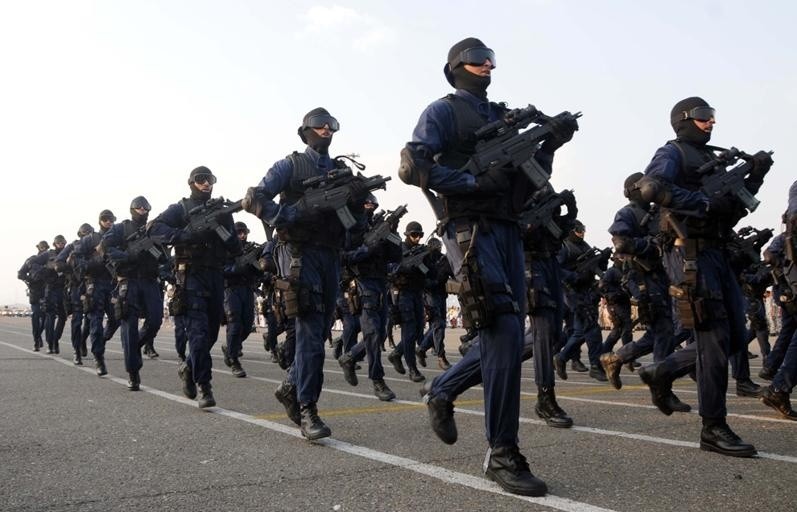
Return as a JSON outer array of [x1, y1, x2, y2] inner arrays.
[[33, 337, 158, 391], [177, 333, 470, 444]]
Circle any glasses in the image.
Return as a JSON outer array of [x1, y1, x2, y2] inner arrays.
[[443, 46, 496, 88], [299, 114, 339, 144], [234, 227, 249, 235], [671, 106, 716, 127], [570, 225, 586, 233], [404, 232, 423, 238], [188, 173, 217, 185], [131, 202, 151, 210]]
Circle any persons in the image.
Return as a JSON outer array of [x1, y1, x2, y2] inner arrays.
[[15, 36, 795, 498]]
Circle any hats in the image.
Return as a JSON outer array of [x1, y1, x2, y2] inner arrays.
[[624, 172, 650, 210], [37, 210, 114, 253], [190, 166, 213, 200], [130, 197, 149, 225], [405, 221, 422, 248], [671, 96, 713, 147], [234, 222, 247, 244], [448, 38, 491, 100], [428, 238, 442, 252], [567, 220, 584, 244], [363, 191, 378, 216], [302, 107, 333, 155]]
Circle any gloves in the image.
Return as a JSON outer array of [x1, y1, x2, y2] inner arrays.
[[476, 174, 510, 197], [386, 215, 399, 234], [175, 231, 196, 244], [348, 180, 368, 208], [542, 118, 573, 154], [215, 212, 232, 235], [749, 151, 771, 181], [602, 248, 611, 265], [560, 190, 578, 219]]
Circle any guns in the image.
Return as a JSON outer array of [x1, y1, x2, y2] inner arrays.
[[608, 231, 671, 303], [234, 241, 300, 300], [285, 169, 392, 231], [662, 149, 774, 240], [458, 104, 583, 189], [562, 247, 614, 295], [364, 205, 408, 256], [40, 240, 180, 298], [446, 278, 482, 328], [182, 197, 247, 258], [401, 242, 449, 287], [740, 227, 793, 301], [339, 269, 358, 290], [521, 190, 573, 239]]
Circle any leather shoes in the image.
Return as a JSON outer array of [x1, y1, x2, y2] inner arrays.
[[484, 445, 548, 496], [638, 365, 674, 415], [700, 419, 758, 457], [534, 385, 573, 429], [624, 362, 635, 372], [600, 351, 624, 390], [589, 364, 608, 381], [553, 354, 568, 381], [572, 360, 589, 372], [670, 390, 692, 412], [635, 362, 641, 367], [736, 351, 797, 420]]
